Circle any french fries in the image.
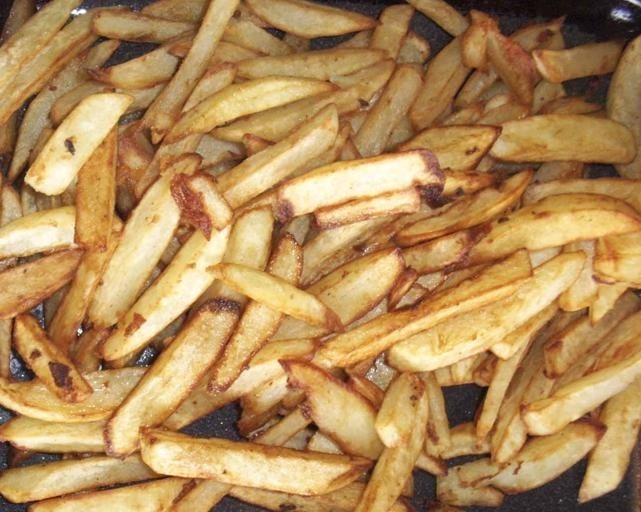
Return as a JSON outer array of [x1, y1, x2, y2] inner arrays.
[[1, 1, 641, 512]]
